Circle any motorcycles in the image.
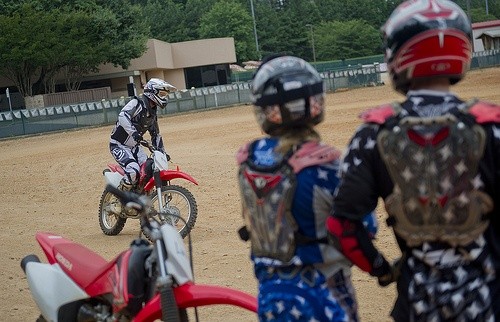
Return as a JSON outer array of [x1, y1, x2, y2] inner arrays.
[[98, 134, 198, 242], [21, 172, 261, 322]]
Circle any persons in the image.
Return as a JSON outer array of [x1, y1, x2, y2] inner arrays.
[[109, 78, 176, 191], [236, 56, 377, 322], [326, 0, 500, 322]]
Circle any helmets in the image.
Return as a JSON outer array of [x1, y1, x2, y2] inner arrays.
[[142, 77, 178, 109], [377, 0, 477, 92], [249, 52, 327, 136]]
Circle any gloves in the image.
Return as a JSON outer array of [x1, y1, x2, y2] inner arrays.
[[132, 130, 144, 143], [377, 254, 404, 288]]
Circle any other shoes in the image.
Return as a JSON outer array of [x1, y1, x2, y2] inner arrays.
[[109, 196, 121, 214]]
